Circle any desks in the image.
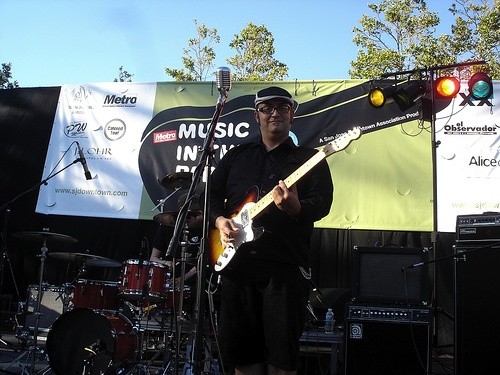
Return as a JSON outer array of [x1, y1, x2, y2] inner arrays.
[[298, 332, 344, 375]]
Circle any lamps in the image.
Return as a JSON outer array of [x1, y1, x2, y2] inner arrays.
[[367, 61, 494, 112]]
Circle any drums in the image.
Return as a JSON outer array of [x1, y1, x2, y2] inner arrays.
[[74, 278, 125, 313], [166, 287, 191, 310], [46, 307, 139, 375], [118, 259, 172, 301]]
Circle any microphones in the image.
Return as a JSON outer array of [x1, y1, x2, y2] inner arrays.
[[141, 237, 146, 257], [216, 67, 232, 110], [76, 142, 92, 180]]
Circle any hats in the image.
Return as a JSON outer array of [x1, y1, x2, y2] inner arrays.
[[253, 86, 295, 107]]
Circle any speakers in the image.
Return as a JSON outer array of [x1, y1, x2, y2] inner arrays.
[[345, 317, 433, 375], [453, 244, 500, 375], [352, 244, 431, 308], [24, 285, 66, 332]]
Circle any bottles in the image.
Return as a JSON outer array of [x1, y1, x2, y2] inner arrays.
[[325, 308, 334, 334]]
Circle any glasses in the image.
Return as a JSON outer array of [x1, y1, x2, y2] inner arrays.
[[255, 104, 293, 115]]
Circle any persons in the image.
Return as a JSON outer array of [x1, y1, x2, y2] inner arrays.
[[199, 87, 334, 375], [150, 211, 203, 319]]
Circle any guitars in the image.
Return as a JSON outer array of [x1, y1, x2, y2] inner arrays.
[[207, 124, 362, 276]]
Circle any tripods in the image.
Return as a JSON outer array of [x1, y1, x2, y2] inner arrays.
[[127, 223, 208, 375], [0, 236, 51, 375]]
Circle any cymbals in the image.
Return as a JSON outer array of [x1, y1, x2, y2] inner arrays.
[[160, 172, 200, 191], [153, 211, 203, 228], [48, 252, 123, 267], [13, 231, 78, 243]]
